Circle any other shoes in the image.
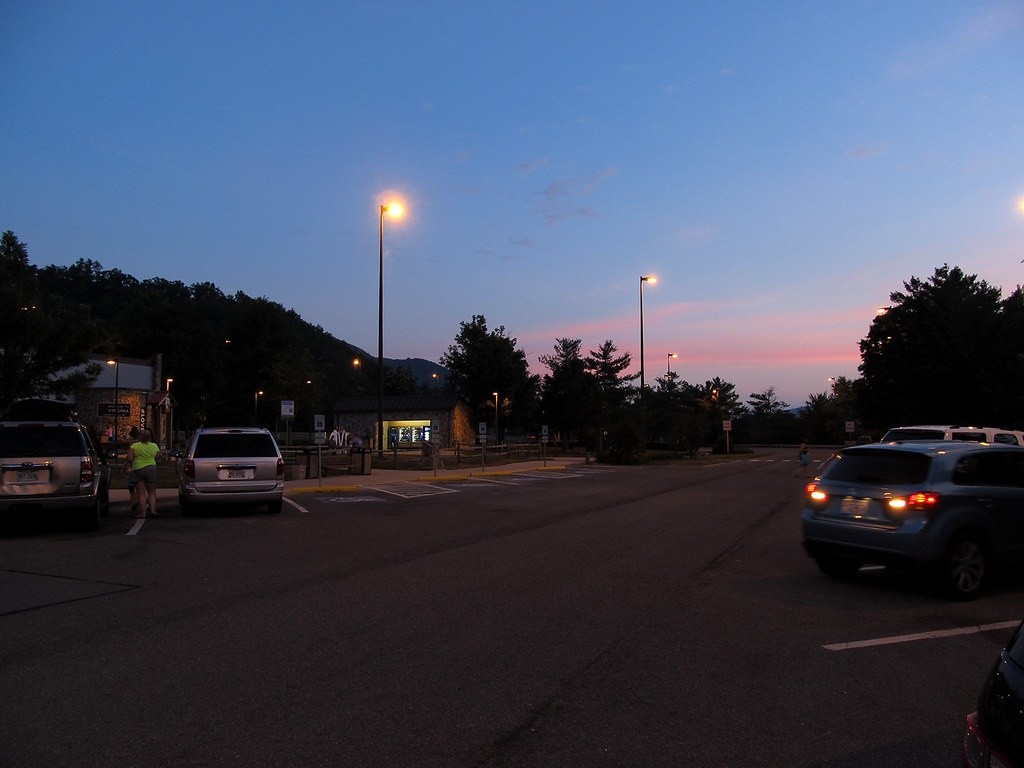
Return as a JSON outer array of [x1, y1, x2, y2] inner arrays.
[[133, 514, 146, 519], [149, 512, 161, 518]]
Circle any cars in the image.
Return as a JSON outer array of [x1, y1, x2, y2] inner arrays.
[[963, 616, 1024, 768]]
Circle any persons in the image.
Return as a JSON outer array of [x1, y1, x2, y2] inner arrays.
[[105, 424, 113, 440], [124, 429, 160, 518], [797, 443, 810, 478]]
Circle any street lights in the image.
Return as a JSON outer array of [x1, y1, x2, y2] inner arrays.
[[378, 201, 406, 457], [493, 392, 498, 444], [668, 353, 677, 374], [107, 358, 119, 450], [640, 275, 657, 392]]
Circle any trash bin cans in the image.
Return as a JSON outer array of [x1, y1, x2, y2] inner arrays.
[[295, 447, 319, 479], [350, 446, 372, 475]]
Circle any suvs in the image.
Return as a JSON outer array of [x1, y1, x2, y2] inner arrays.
[[169, 426, 285, 515], [0, 421, 113, 529], [799, 425, 1024, 597]]
